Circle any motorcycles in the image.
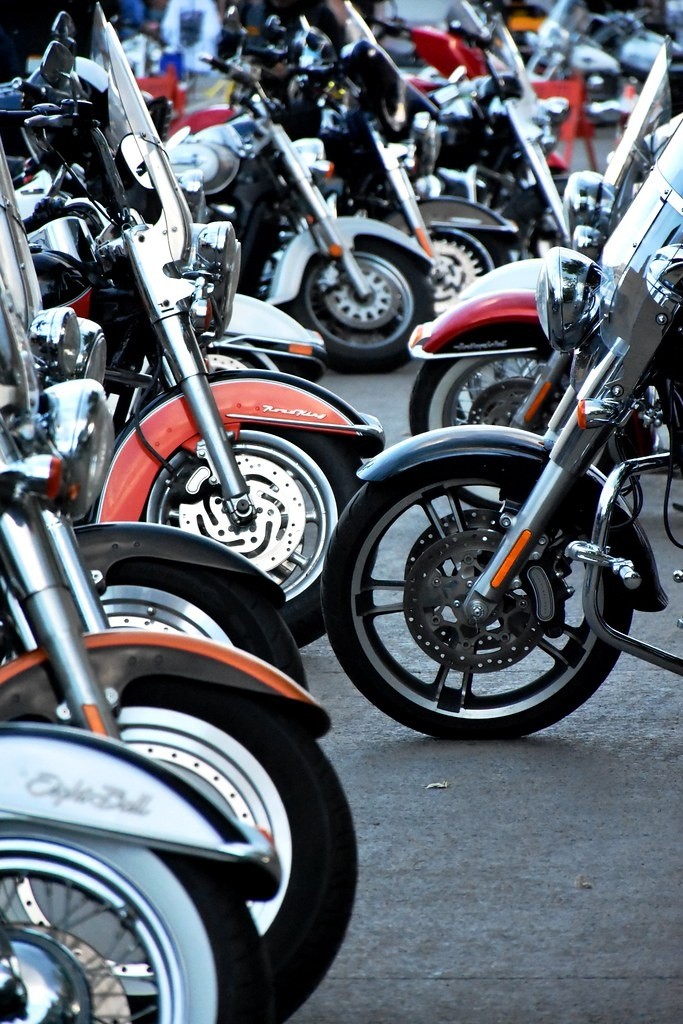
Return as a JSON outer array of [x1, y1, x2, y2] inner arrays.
[[0, 0, 683, 1024]]
